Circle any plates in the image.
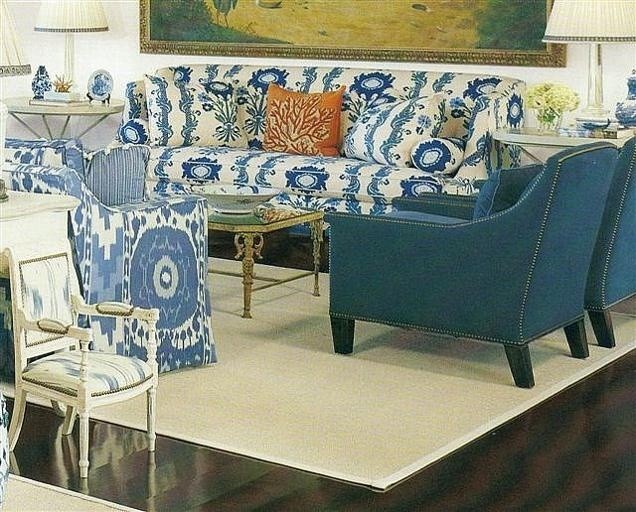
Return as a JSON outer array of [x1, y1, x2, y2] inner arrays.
[[86, 69, 113, 101]]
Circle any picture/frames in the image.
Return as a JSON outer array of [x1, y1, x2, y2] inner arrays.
[[139, 0, 568, 69]]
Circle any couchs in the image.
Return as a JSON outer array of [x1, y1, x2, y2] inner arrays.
[[107, 64, 526, 245], [2, 137, 218, 377]]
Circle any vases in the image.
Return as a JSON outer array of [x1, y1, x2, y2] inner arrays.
[[537, 107, 563, 132]]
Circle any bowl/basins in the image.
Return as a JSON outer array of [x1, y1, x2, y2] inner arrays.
[[190, 182, 283, 216]]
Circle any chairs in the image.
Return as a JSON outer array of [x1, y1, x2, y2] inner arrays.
[[7, 238, 161, 476], [322, 138, 636, 390]]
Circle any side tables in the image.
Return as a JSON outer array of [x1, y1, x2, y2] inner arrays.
[[2, 95, 125, 152]]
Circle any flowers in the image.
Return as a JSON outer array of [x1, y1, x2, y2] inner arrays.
[[526, 81, 580, 129]]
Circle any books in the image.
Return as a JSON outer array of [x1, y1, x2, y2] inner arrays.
[[29, 99, 88, 108], [561, 129, 618, 139]]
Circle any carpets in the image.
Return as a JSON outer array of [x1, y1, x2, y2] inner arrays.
[[1, 257, 636, 491]]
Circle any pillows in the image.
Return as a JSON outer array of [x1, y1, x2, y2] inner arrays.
[[343, 93, 448, 168], [144, 73, 249, 150], [263, 80, 345, 158]]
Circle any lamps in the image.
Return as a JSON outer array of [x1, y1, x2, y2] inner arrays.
[[541, 0, 636, 124], [29, 2, 109, 108]]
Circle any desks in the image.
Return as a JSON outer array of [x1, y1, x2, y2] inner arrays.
[[492, 130, 636, 166], [203, 203, 325, 318]]
